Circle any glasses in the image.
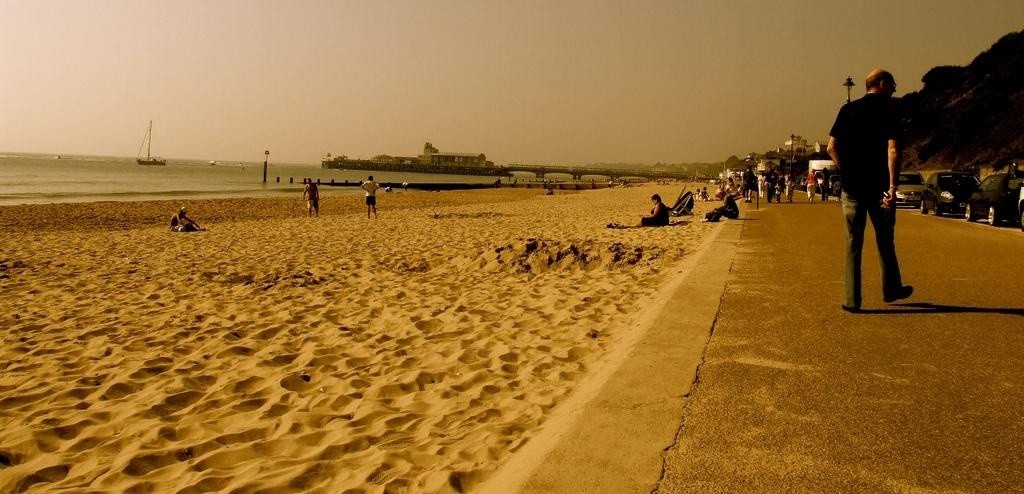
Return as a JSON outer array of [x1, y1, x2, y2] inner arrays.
[[890, 83, 896, 89]]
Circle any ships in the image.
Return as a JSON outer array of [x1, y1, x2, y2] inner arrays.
[[320, 140, 512, 176]]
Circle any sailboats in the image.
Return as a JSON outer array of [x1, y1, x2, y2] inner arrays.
[[135, 118, 167, 165]]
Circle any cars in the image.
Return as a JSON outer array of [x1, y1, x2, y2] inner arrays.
[[798, 176, 807, 191]]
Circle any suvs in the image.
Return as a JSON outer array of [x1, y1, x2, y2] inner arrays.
[[895, 171, 1024, 231]]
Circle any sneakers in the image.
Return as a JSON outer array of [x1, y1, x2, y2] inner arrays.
[[842, 304, 860, 313], [617, 225, 625, 229], [883, 286, 913, 302], [744, 196, 829, 204]]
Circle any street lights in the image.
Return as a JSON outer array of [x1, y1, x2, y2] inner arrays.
[[841, 74, 855, 103], [789, 133, 794, 176]]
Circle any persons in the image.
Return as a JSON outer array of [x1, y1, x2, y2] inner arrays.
[[302, 177, 320, 218], [591, 177, 597, 189], [494, 177, 501, 188], [361, 175, 379, 220], [385, 185, 392, 193], [703, 190, 739, 222], [169, 206, 206, 232], [631, 195, 669, 227], [827, 67, 914, 312], [608, 179, 613, 189], [403, 181, 409, 192], [545, 188, 552, 195], [693, 165, 842, 204]]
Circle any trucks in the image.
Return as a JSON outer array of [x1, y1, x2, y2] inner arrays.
[[808, 159, 836, 193]]
